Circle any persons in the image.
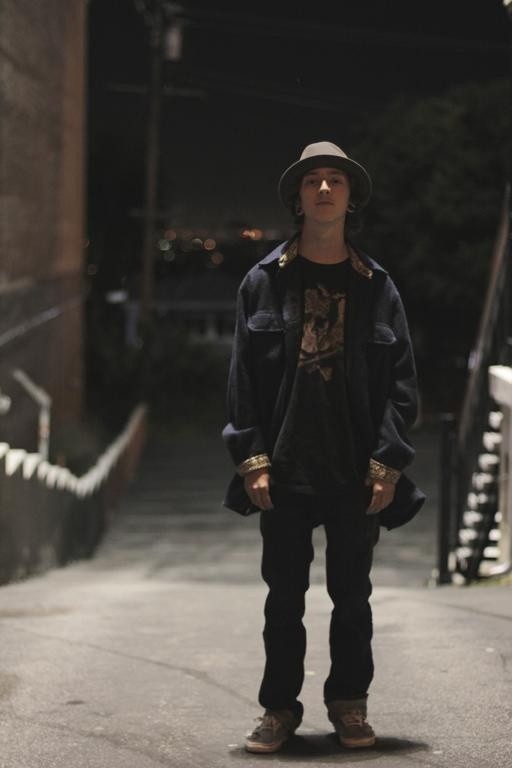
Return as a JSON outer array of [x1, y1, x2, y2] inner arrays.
[[222, 140, 426, 754]]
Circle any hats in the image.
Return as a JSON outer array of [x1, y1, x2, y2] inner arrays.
[[277, 141, 371, 211]]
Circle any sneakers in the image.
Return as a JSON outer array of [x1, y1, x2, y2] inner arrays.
[[247, 702, 302, 753], [326, 706, 379, 748]]
